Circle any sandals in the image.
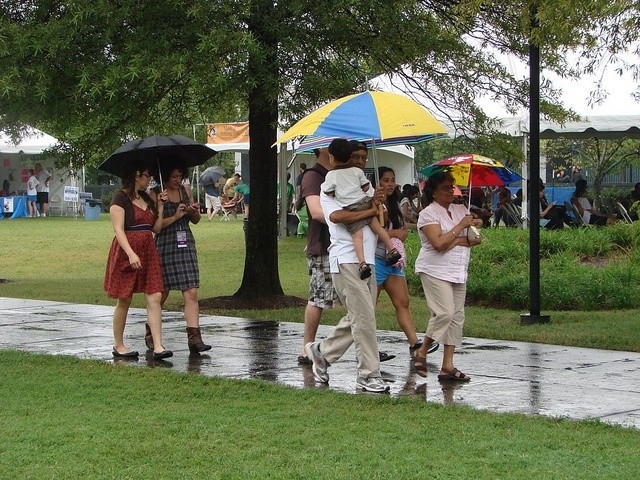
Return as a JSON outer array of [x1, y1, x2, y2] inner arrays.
[[359, 262, 371, 280], [386, 248, 401, 266], [410, 348, 428, 378], [378, 350, 396, 362], [437, 368, 471, 382], [297, 355, 313, 366]]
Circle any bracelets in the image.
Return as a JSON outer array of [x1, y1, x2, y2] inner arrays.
[[452, 230, 456, 236]]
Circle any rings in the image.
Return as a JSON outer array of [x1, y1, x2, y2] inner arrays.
[[383, 195, 385, 198]]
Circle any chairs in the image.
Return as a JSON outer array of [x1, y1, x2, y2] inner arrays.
[[571, 202, 587, 227], [218, 196, 232, 221], [49, 194, 63, 215], [599, 195, 615, 214], [66, 201, 78, 217], [512, 203, 522, 225], [505, 202, 522, 227], [616, 201, 633, 223], [564, 200, 577, 224], [516, 206, 531, 225], [501, 204, 517, 225]]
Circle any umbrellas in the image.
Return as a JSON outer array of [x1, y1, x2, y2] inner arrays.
[[96, 135, 219, 203], [270, 76, 450, 230], [418, 154, 526, 216], [199, 166, 225, 186]]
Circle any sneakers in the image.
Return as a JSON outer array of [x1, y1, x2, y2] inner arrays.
[[356, 375, 390, 392], [304, 341, 329, 383], [409, 341, 439, 358]]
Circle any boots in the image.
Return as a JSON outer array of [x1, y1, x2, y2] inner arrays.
[[186, 326, 211, 354], [144, 322, 154, 350]]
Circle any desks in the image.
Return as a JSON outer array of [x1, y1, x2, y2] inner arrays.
[[0, 195, 27, 217]]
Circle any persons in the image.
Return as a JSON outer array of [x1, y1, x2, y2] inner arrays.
[[323, 139, 401, 279], [578, 190, 616, 225], [557, 171, 570, 183], [506, 189, 522, 226], [223, 173, 243, 218], [145, 157, 212, 354], [298, 147, 396, 364], [571, 166, 584, 183], [371, 166, 439, 362], [570, 179, 587, 215], [228, 182, 250, 221], [304, 139, 391, 393], [34, 163, 52, 218], [25, 169, 39, 218], [411, 172, 481, 381], [492, 185, 511, 227], [618, 182, 640, 222], [538, 186, 563, 230], [399, 184, 419, 224], [295, 162, 307, 196], [103, 162, 174, 360], [204, 180, 222, 222], [285, 172, 295, 212]]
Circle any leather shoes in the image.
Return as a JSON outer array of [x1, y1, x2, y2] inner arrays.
[[112, 346, 139, 358], [153, 349, 173, 360]]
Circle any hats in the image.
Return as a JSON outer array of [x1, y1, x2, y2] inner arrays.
[[350, 140, 368, 151]]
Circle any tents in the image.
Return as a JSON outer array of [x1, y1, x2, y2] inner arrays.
[[519, 115, 640, 229]]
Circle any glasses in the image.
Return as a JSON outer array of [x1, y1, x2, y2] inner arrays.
[[137, 172, 150, 178], [349, 153, 369, 163]]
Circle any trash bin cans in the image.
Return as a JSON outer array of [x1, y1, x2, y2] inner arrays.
[[85, 199, 103, 220]]
